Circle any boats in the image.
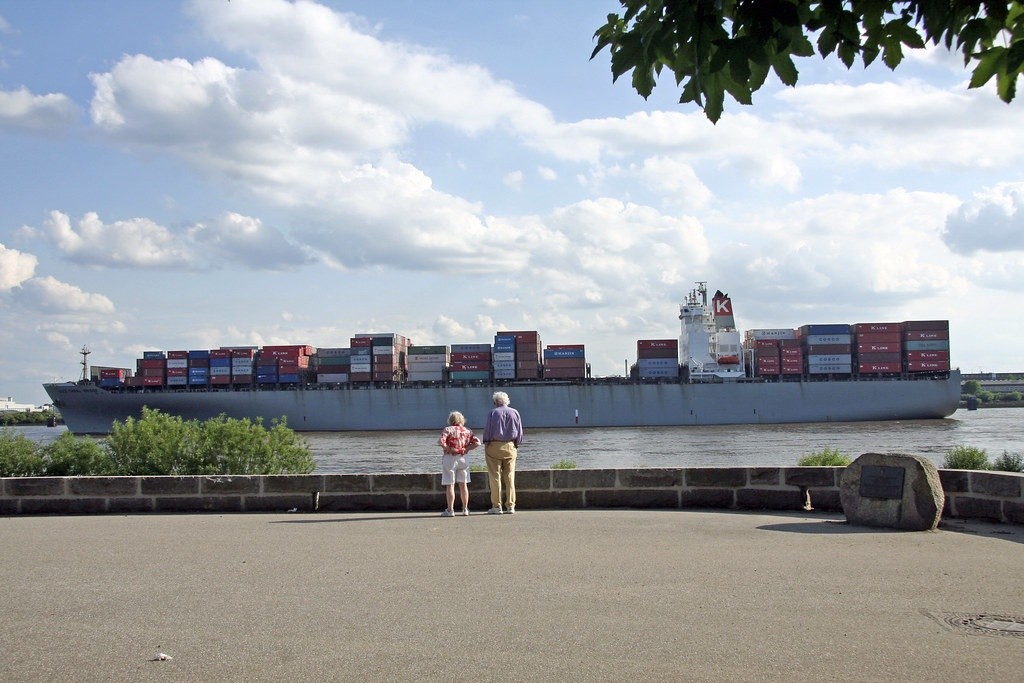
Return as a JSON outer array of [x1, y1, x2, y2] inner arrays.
[[47, 414, 57, 427], [966, 397, 978, 412]]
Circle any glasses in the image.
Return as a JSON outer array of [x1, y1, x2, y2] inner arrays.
[[494, 402, 496, 405]]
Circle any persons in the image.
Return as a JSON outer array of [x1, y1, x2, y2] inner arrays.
[[482, 391, 523, 515], [437, 411, 481, 517]]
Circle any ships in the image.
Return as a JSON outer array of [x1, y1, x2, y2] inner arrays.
[[42, 282, 962, 436]]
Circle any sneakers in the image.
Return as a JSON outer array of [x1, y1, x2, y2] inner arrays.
[[463, 508, 470, 516], [441, 509, 455, 516], [507, 507, 515, 513], [488, 506, 503, 514]]
